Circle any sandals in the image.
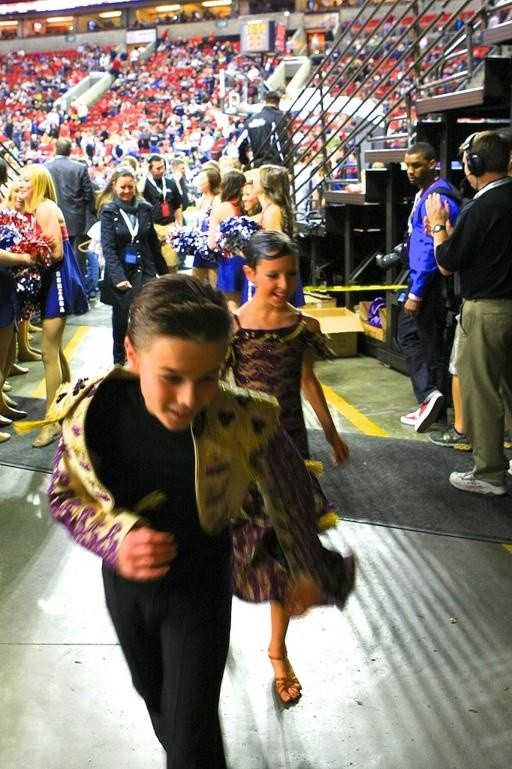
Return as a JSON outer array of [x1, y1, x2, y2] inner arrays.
[[264, 646, 305, 708]]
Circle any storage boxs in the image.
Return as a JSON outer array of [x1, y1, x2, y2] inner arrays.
[[292, 306, 366, 359]]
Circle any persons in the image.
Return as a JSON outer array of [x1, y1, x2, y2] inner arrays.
[[39, 272, 318, 768], [227, 230, 349, 706]]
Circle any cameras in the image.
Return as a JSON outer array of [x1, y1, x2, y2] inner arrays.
[[375, 243, 410, 271]]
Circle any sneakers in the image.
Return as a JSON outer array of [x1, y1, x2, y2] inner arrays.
[[16, 320, 43, 361], [32, 421, 63, 450], [0, 362, 29, 444], [502, 432, 512, 449], [428, 424, 473, 453], [413, 389, 445, 434], [399, 407, 422, 427], [447, 466, 508, 496]]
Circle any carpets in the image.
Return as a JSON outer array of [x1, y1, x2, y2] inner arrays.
[[0, 390, 511, 547]]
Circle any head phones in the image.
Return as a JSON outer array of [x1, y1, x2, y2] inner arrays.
[[146, 155, 167, 170], [463, 131, 486, 178]]
